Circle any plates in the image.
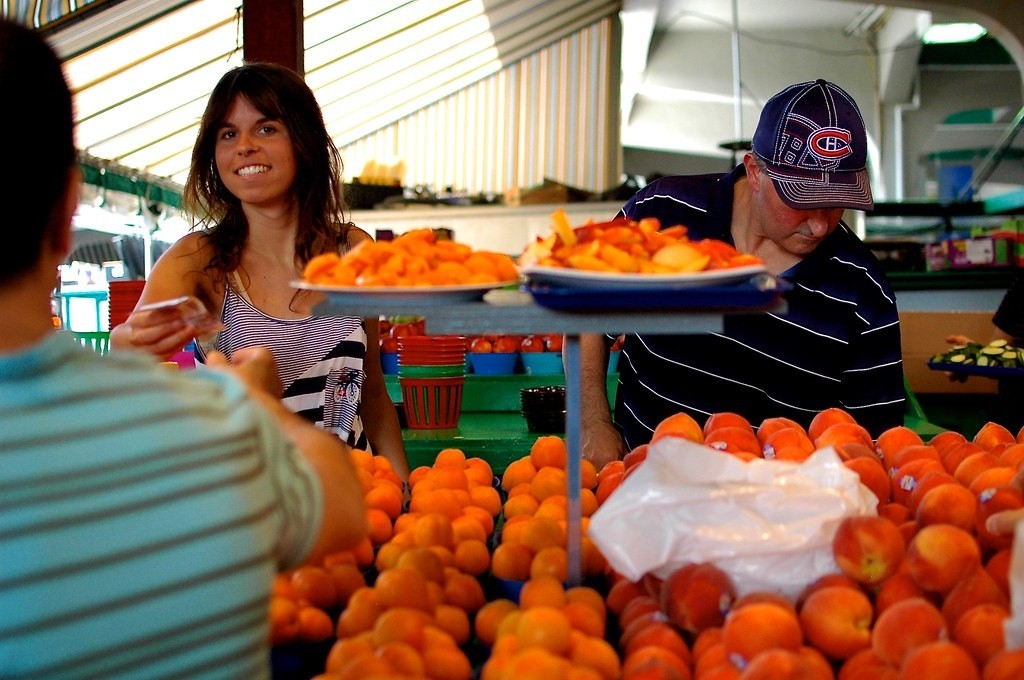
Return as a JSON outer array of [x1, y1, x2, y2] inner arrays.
[[288, 267, 530, 305], [520, 257, 770, 290]]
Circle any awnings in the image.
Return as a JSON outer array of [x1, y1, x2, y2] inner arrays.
[[2, 0, 622, 208]]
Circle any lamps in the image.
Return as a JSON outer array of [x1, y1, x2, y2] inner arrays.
[[916, 10, 988, 44]]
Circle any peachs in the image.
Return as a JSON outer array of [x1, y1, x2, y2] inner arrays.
[[304, 211, 764, 288], [263, 406, 1023, 680]]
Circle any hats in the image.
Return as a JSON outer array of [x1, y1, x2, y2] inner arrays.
[[750, 80, 876, 211]]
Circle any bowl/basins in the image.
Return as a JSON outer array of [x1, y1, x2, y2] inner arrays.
[[520, 382, 569, 433]]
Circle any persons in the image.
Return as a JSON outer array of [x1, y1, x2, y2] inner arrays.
[[109, 66, 413, 519], [2, 12, 368, 671], [577, 77, 912, 474], [923, 263, 1024, 402]]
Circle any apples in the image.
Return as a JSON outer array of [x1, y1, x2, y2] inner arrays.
[[377, 320, 562, 355]]
